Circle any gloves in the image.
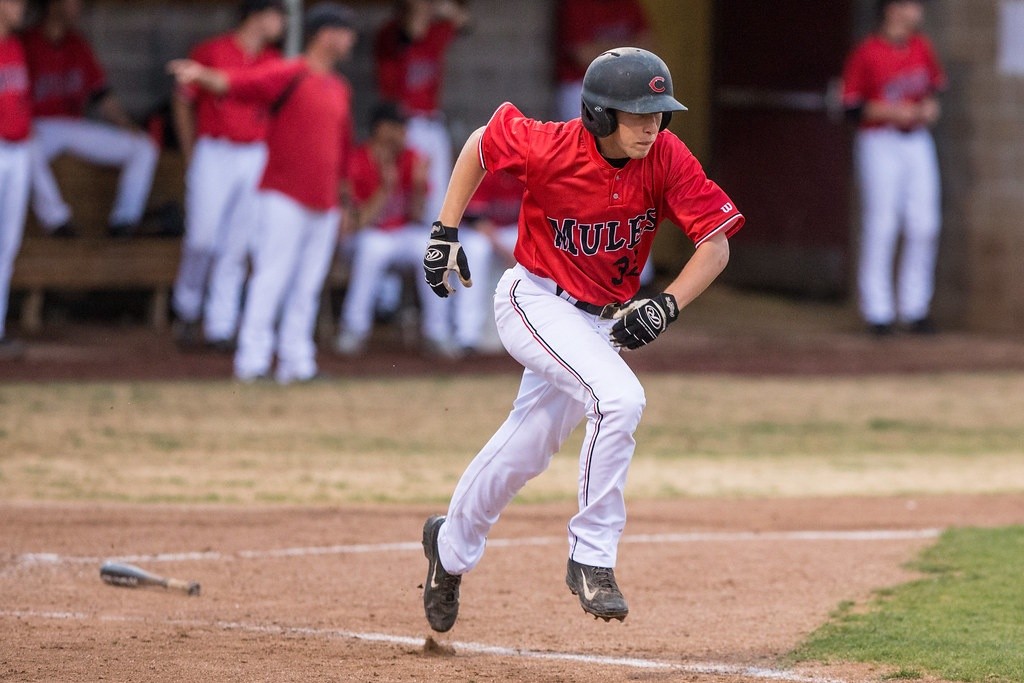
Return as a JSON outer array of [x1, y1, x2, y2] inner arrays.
[[423, 221, 472, 298], [609, 293, 680, 352]]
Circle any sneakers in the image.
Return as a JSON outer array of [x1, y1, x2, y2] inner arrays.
[[565, 557, 629, 622], [423, 514, 462, 633]]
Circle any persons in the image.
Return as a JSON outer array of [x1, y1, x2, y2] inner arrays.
[[422, 46, 745, 634], [840, 0, 946, 332], [0, 0, 651, 389]]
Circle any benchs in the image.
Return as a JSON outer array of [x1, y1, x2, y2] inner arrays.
[[10, 154, 355, 346]]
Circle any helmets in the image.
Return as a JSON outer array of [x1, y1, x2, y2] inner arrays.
[[581, 47, 688, 138]]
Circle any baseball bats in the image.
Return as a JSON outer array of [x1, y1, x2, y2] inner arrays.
[[99, 561, 203, 600]]
